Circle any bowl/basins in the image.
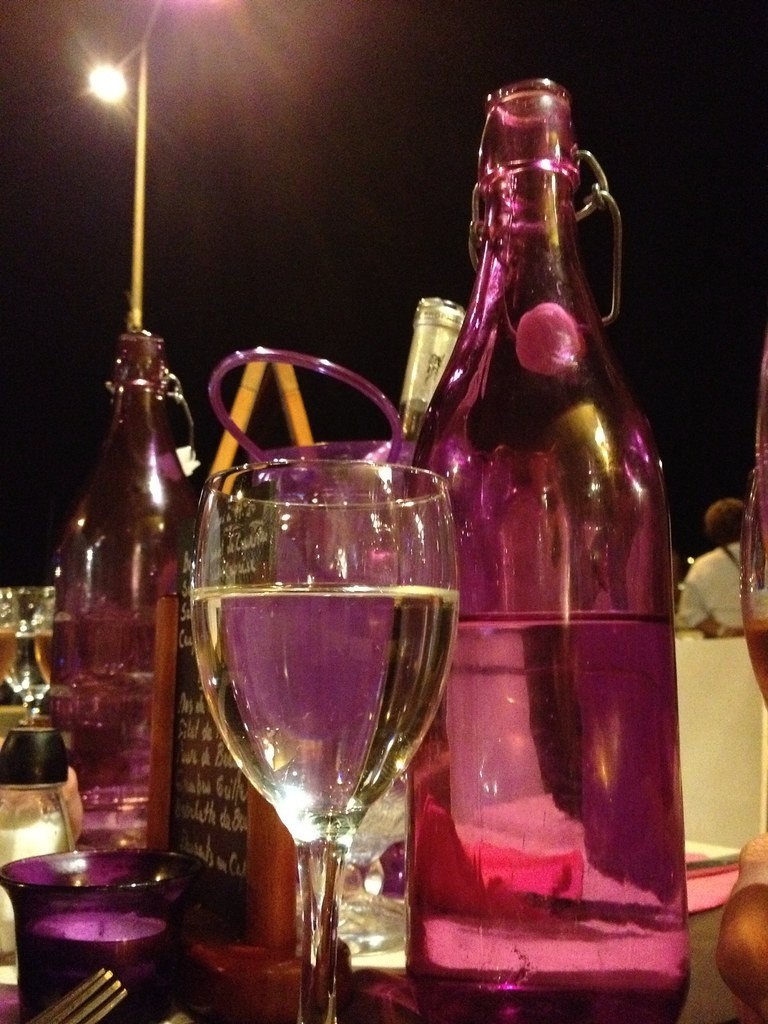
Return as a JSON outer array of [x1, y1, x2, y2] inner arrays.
[[0, 727, 70, 791]]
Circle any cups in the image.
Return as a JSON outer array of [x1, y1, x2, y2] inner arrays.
[[737, 343, 768, 707], [0, 848, 208, 1023]]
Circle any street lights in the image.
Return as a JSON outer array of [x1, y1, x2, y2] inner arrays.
[[87, 1, 172, 339]]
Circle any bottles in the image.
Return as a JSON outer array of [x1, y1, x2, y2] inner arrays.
[[51, 325, 191, 858], [0, 766, 84, 985], [399, 297, 469, 440], [403, 79, 692, 1023]]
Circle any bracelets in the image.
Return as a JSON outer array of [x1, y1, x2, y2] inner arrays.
[[717, 626, 726, 637]]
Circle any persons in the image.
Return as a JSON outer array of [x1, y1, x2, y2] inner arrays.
[[715, 832, 768, 1024], [677, 499, 768, 639]]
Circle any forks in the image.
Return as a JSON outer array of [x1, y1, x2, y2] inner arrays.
[[30, 969, 128, 1024]]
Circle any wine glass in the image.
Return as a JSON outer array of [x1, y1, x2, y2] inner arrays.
[[191, 459, 462, 1023], [294, 774, 407, 966], [0, 586, 55, 726]]
